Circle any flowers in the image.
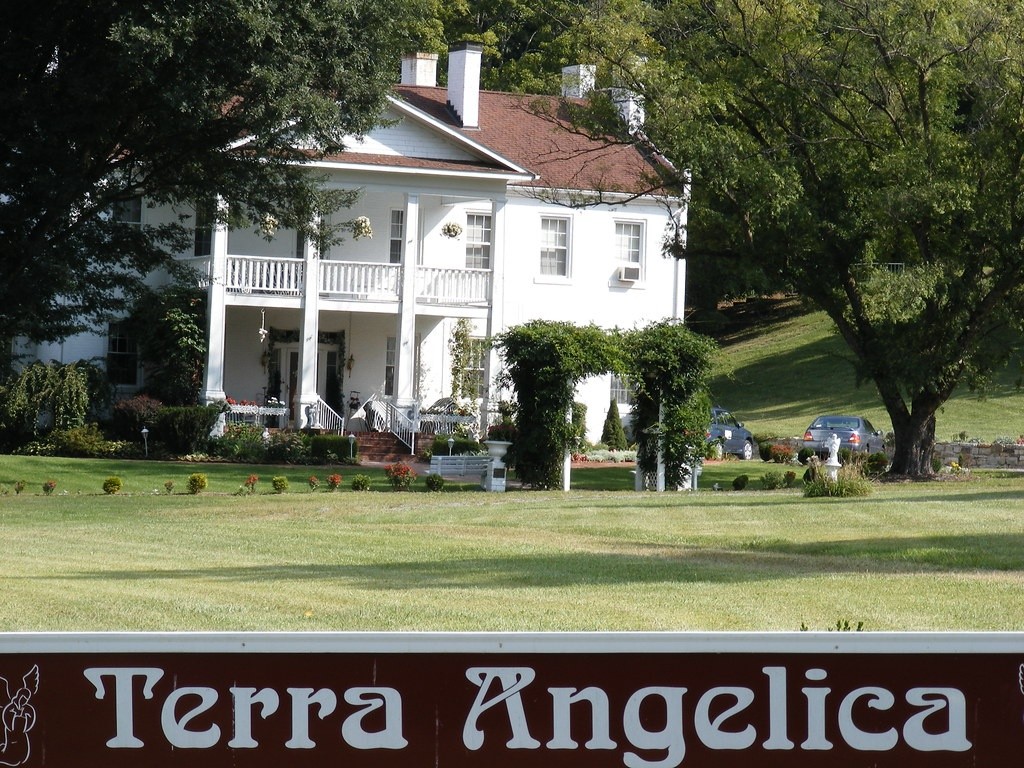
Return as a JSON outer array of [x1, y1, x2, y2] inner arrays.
[[226, 398, 256, 406], [442, 224, 462, 239], [262, 213, 278, 236], [355, 216, 375, 240], [258, 397, 285, 408], [419, 408, 472, 416], [487, 425, 515, 443]]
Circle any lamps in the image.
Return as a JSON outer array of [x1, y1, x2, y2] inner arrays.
[[262, 350, 268, 376], [447, 436, 455, 456], [348, 431, 355, 458], [347, 354, 355, 378], [140, 426, 149, 458]]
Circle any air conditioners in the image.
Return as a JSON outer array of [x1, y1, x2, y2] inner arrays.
[[618, 267, 640, 282]]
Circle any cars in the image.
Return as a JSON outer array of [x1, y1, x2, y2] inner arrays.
[[803, 415, 888, 466]]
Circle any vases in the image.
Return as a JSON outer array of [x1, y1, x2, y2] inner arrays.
[[482, 439, 513, 461], [419, 414, 476, 423], [259, 407, 286, 416], [229, 404, 258, 414]]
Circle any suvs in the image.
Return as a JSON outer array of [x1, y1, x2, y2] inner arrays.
[[706, 409, 754, 459]]
